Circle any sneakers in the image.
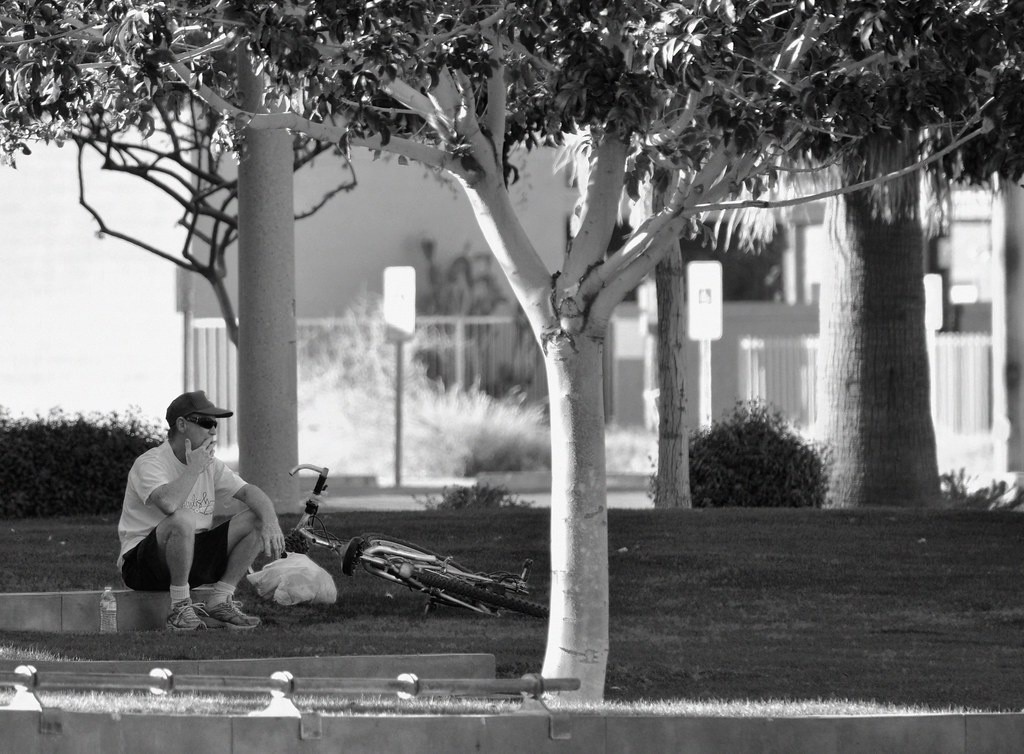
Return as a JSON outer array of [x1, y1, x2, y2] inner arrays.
[[197, 599, 262, 629], [166, 598, 208, 633]]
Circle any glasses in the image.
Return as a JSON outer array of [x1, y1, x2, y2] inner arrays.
[[185, 417, 217, 429]]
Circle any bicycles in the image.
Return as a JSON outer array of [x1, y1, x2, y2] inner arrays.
[[250, 462, 551, 621]]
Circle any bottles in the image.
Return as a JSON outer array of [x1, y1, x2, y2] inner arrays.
[[99, 586, 118, 634]]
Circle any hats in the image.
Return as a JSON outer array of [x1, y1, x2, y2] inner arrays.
[[166, 390, 234, 427]]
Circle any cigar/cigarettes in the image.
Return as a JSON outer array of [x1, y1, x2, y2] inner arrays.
[[211, 440, 217, 444]]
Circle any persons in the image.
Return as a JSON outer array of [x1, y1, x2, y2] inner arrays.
[[117, 390, 285, 634]]
